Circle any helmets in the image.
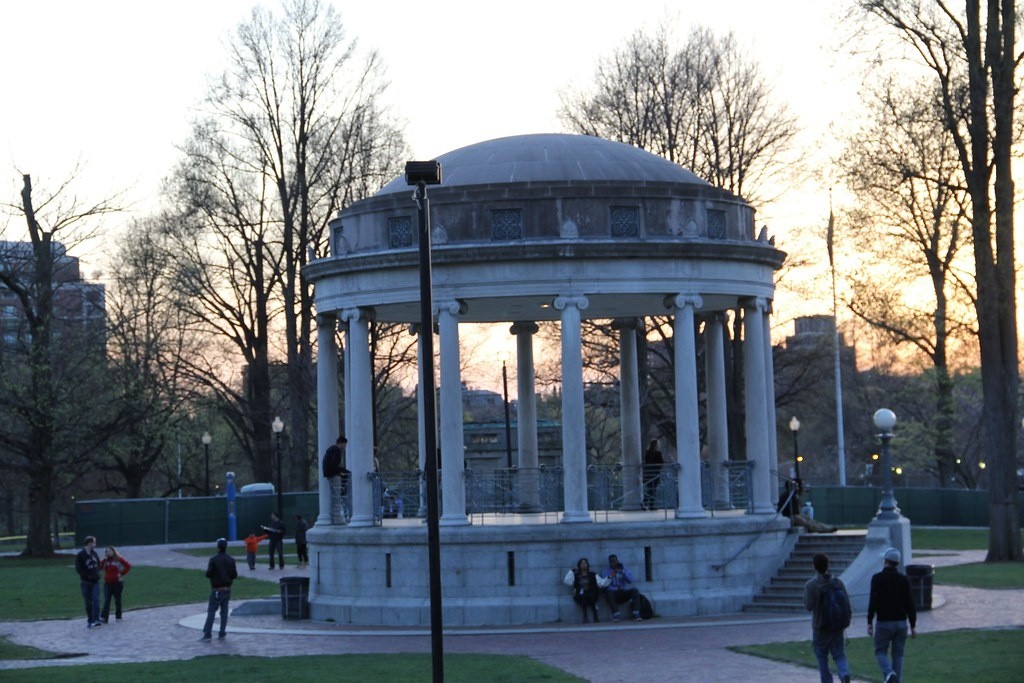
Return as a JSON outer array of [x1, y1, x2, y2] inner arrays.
[[880, 548, 900, 563], [217, 538, 227, 547]]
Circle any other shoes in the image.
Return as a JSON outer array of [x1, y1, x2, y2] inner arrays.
[[633, 615, 643, 621], [219, 631, 226, 640], [91, 622, 101, 628], [612, 618, 620, 623], [884, 671, 897, 683], [197, 634, 211, 642], [842, 675, 850, 683]]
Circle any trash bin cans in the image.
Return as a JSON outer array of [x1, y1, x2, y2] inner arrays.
[[906, 564, 934, 611], [280, 576, 309, 619]]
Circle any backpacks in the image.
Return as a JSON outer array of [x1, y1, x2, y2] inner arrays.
[[816, 578, 850, 632], [630, 594, 653, 619]]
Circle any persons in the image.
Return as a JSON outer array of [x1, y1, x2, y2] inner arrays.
[[803, 552, 852, 683], [197, 538, 238, 642], [563, 558, 616, 623], [641, 440, 665, 511], [99, 546, 132, 623], [266, 512, 286, 570], [600, 555, 642, 622], [75, 536, 102, 628], [245, 531, 269, 570], [778, 478, 837, 533], [867, 548, 918, 683], [322, 436, 348, 525], [373, 447, 405, 518], [294, 514, 309, 565]]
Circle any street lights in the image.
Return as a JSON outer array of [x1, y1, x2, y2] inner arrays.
[[272, 417, 284, 518], [790, 416, 801, 493], [405, 159, 445, 683], [201, 432, 211, 496], [178, 413, 194, 496]]
